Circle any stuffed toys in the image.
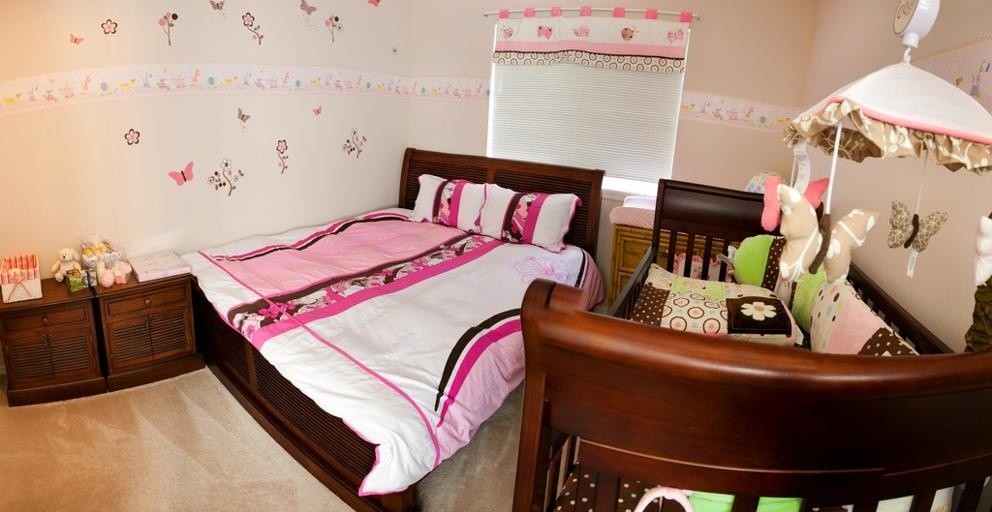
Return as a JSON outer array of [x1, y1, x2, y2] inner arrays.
[[49, 234, 132, 287]]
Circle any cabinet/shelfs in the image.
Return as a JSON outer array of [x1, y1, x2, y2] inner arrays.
[[0, 266, 205, 407], [609, 224, 738, 312]]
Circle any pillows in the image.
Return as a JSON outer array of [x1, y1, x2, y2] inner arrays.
[[412, 173, 583, 254], [625, 263, 919, 357]]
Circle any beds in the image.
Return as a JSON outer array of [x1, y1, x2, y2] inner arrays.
[[193, 148, 605, 511], [520, 177, 991, 512]]
